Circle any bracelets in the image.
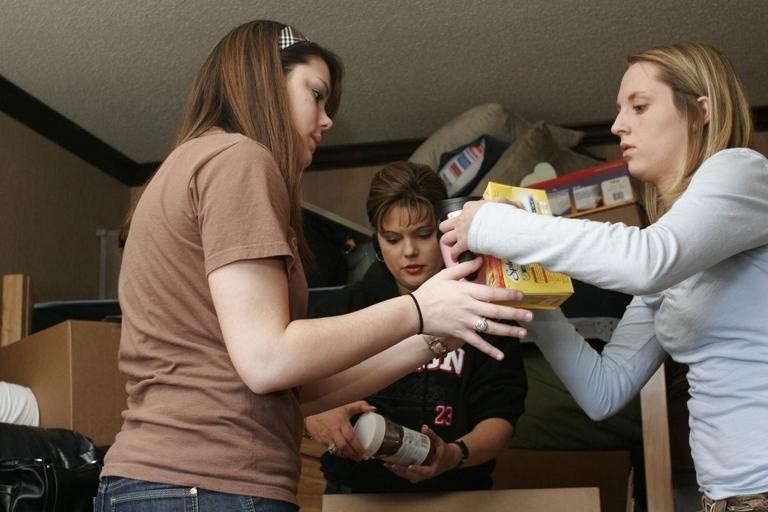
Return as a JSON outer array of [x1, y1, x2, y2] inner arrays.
[[406, 291, 424, 337], [450, 439, 469, 470], [421, 333, 448, 359]]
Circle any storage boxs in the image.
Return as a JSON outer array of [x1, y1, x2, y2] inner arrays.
[[0, 318, 130, 447]]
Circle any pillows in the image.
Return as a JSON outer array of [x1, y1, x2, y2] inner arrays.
[[407, 99, 607, 198]]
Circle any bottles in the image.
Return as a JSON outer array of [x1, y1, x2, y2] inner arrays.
[[349, 413, 439, 472]]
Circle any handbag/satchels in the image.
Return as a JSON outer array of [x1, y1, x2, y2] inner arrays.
[[0, 425, 97, 512]]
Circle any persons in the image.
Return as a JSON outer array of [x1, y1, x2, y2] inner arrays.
[[91, 19, 536, 512], [437, 41, 767, 510], [300, 159, 529, 502]]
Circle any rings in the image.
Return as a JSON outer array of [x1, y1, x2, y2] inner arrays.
[[326, 445, 337, 454], [474, 316, 488, 333]]
